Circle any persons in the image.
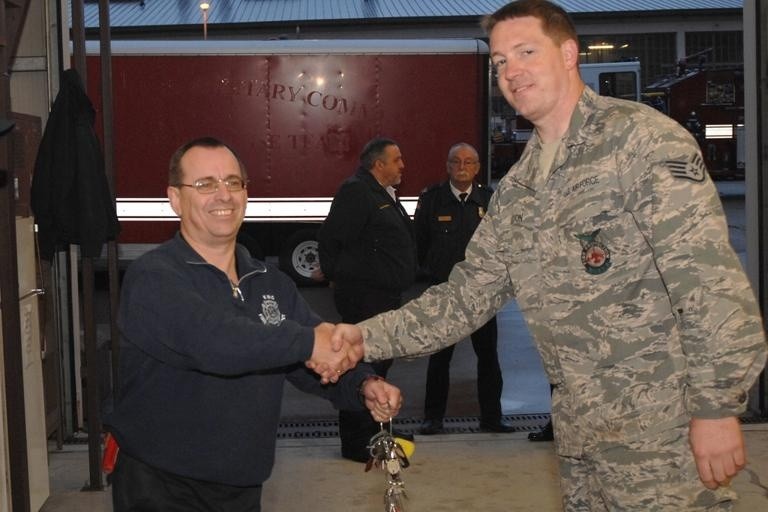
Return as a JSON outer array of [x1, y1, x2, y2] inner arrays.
[[317, 137, 422, 464], [412, 141, 517, 435], [104, 137, 405, 512], [329, 0, 768, 512], [528, 385, 554, 442]]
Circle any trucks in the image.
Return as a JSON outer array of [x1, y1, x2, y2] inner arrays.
[[67, 37, 492, 290], [491, 63, 641, 163]]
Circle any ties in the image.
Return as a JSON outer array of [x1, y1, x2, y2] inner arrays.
[[459, 193, 468, 207]]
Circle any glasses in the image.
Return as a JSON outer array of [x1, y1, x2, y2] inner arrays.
[[173, 176, 245, 194]]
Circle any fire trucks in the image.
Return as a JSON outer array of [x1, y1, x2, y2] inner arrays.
[[619, 48, 745, 180]]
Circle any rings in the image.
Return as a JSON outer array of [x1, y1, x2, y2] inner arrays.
[[336, 369, 345, 373]]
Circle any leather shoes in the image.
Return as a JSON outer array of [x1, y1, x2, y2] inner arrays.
[[527, 417, 555, 441], [482, 417, 515, 433], [341, 427, 414, 467], [420, 414, 443, 433]]
